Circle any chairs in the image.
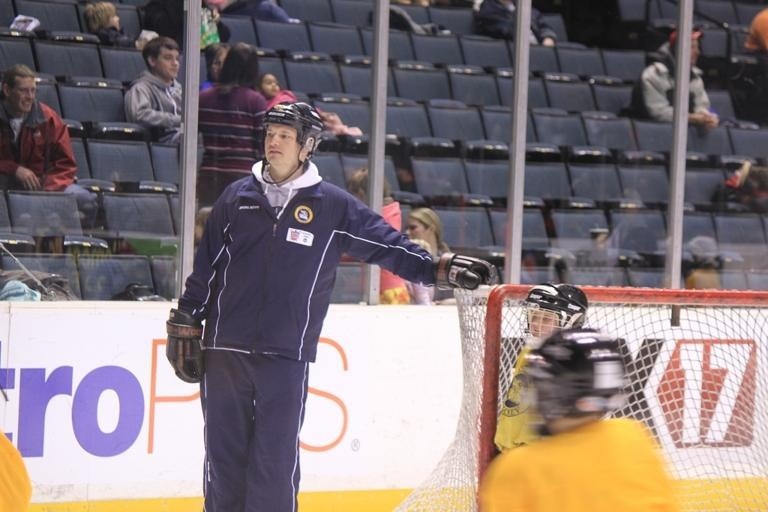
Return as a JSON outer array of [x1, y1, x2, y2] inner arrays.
[[0, 0, 767, 301]]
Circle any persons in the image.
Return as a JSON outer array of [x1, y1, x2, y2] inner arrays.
[[345, 164, 407, 308], [84, 0, 303, 56], [474, 1, 565, 48], [125, 37, 363, 135], [738, 7, 768, 57], [492, 282, 593, 453], [165, 101, 498, 511], [638, 23, 718, 131], [403, 207, 459, 305], [480, 326, 693, 512], [193, 42, 267, 210], [0, 65, 98, 229]]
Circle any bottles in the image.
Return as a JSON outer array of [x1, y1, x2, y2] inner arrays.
[[721, 159, 751, 188]]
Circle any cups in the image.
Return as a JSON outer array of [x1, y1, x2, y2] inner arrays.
[[707, 109, 718, 126], [589, 227, 609, 255]]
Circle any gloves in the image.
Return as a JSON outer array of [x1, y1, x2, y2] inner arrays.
[[164, 308, 204, 382], [433, 251, 497, 291]]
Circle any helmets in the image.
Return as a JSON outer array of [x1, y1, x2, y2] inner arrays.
[[522, 330, 627, 436], [522, 283, 588, 351], [265, 103, 324, 156]]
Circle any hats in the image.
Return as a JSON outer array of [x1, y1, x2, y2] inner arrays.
[[668, 30, 702, 48]]
